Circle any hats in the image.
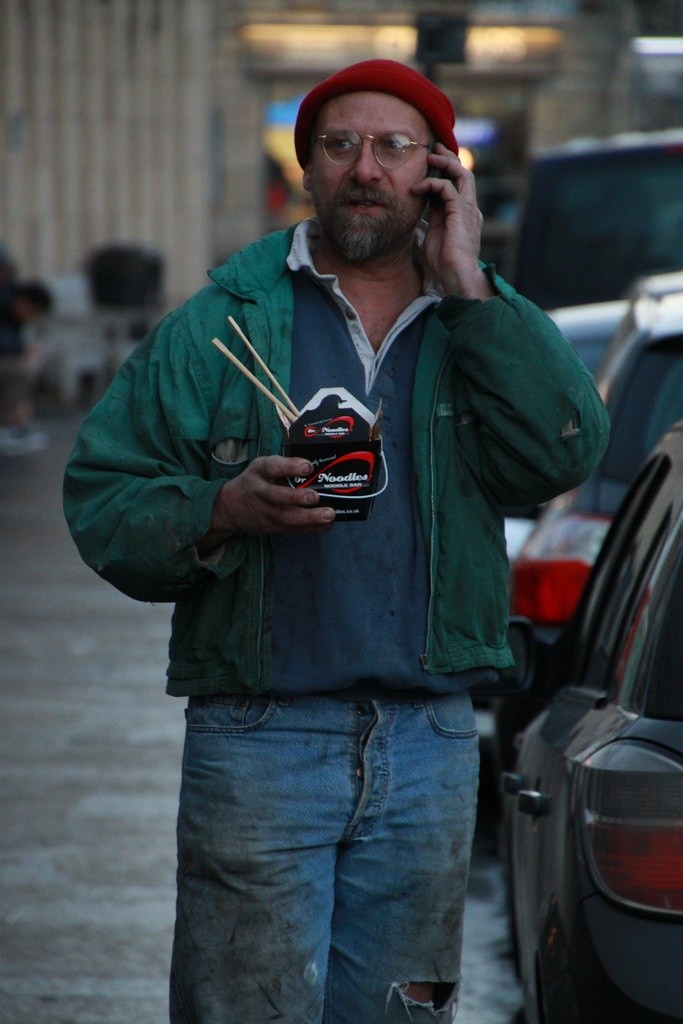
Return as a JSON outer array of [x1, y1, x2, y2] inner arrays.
[[293, 58, 458, 172]]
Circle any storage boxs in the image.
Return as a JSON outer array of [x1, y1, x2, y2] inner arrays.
[[274, 385, 387, 522]]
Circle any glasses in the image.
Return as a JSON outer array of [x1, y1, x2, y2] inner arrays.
[[309, 129, 433, 169]]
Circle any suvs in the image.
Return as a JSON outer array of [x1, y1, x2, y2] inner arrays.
[[508, 270, 682, 626], [501, 126, 682, 382]]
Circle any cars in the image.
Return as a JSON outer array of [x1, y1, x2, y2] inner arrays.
[[471, 295, 629, 749], [495, 424, 682, 1024]]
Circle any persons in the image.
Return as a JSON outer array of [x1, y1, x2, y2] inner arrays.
[[0, 282, 51, 450], [63, 59, 610, 1024]]
